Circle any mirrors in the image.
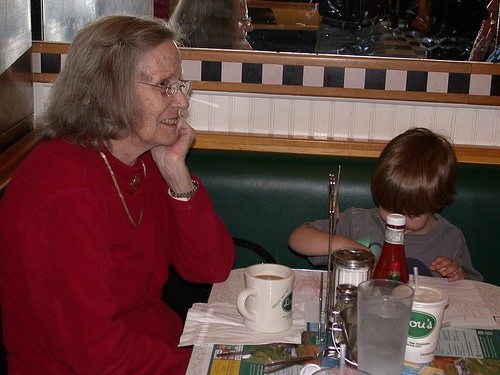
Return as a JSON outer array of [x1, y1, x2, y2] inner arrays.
[[43, 0, 500, 64]]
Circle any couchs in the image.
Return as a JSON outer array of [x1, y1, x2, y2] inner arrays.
[[0, 147, 500, 375]]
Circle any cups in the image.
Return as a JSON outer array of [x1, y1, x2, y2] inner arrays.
[[392, 284, 449, 364], [358, 279, 415, 375], [237, 263, 295, 334]]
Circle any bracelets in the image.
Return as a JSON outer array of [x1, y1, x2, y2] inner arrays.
[[169, 179, 200, 199]]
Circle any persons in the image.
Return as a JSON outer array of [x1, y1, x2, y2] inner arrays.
[[167, 0, 254, 51], [0, 15, 237, 375], [285, 126, 484, 282], [314, 0, 500, 64]]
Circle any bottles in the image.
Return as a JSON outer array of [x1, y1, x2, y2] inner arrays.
[[328, 247, 375, 323], [334, 283, 358, 364], [370, 214, 410, 296]]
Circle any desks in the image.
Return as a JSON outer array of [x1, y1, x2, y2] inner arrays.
[[185, 267, 500, 375]]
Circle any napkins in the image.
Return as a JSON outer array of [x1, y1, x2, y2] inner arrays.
[[177, 302, 308, 346]]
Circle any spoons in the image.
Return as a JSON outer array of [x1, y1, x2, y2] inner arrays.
[[216, 342, 297, 357]]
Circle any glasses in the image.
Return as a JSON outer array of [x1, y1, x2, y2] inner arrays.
[[234, 17, 251, 27], [134, 80, 192, 98]]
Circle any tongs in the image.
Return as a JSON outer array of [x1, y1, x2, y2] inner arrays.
[[262, 351, 327, 374]]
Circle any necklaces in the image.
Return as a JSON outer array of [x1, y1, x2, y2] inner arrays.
[[92, 135, 148, 229]]
